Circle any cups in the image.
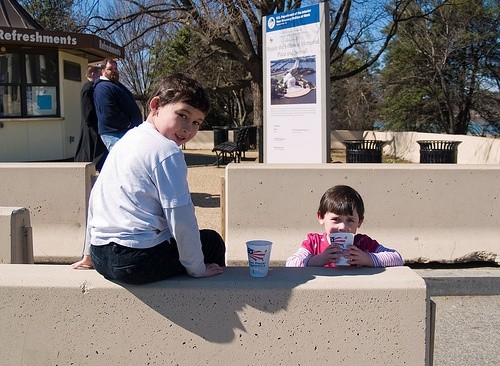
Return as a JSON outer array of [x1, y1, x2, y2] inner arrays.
[[327, 232, 355, 267], [246, 240, 273, 277]]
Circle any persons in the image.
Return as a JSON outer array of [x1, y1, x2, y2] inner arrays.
[[73, 62, 107, 162], [72, 73, 226, 285], [286, 185, 405, 268], [91, 57, 143, 152]]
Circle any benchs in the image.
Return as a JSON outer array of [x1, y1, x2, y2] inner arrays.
[[212, 127, 250, 167]]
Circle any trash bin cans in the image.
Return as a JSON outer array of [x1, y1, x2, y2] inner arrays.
[[213, 126, 229, 147], [341, 138, 383, 163], [417, 140, 463, 164]]
